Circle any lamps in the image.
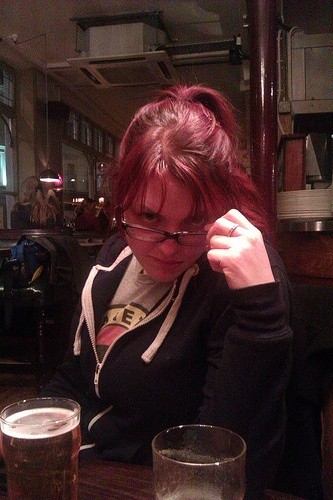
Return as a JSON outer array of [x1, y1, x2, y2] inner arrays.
[[38, 35, 60, 182]]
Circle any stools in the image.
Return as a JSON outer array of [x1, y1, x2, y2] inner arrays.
[[0, 235, 64, 395]]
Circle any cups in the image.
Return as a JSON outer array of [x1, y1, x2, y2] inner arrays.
[[151, 424, 247, 500], [0, 395, 81, 500]]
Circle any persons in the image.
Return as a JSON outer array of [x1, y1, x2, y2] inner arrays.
[[10, 170, 108, 235], [39, 83, 303, 500]]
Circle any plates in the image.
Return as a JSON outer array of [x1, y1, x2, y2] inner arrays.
[[276, 188, 333, 218]]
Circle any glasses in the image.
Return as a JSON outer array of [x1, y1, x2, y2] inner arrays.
[[118, 209, 212, 247]]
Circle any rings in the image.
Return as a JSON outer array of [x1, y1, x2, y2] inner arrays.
[[227, 223, 238, 237]]
